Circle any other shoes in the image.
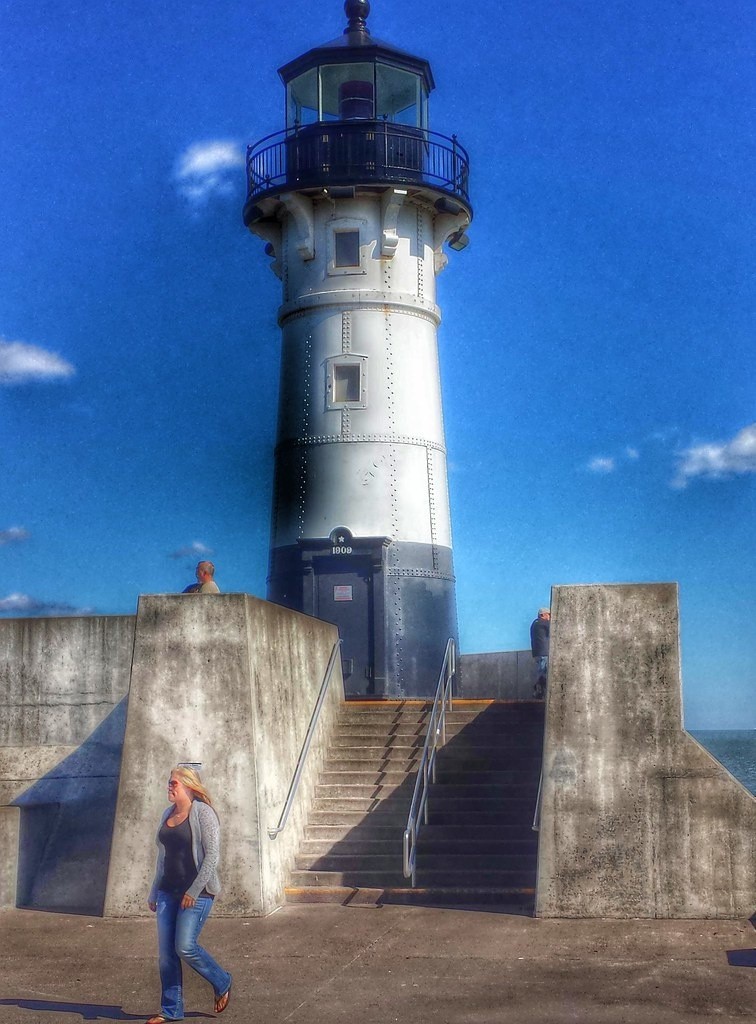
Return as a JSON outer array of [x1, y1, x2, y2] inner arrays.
[[531, 684, 544, 698]]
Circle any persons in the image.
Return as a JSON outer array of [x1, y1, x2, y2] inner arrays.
[[530, 608, 550, 699], [183, 561, 220, 593], [147, 766, 233, 1024]]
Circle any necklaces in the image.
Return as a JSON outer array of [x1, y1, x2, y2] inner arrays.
[[174, 809, 183, 818]]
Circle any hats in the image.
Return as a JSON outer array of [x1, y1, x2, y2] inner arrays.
[[538, 608, 551, 615]]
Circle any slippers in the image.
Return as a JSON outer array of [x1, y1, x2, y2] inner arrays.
[[213, 986, 232, 1012], [144, 1014, 174, 1024]]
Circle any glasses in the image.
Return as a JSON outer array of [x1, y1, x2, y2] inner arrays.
[[168, 780, 181, 789]]
